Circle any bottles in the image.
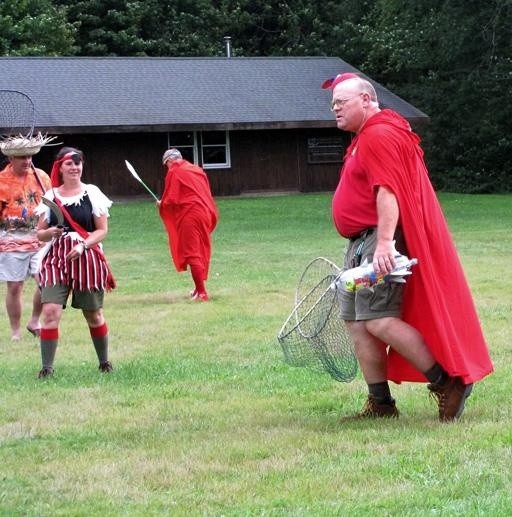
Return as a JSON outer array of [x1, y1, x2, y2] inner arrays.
[[330, 256, 412, 293]]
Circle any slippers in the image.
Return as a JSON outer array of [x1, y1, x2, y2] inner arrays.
[[26, 326, 41, 337]]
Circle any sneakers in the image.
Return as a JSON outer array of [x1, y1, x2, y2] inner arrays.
[[190, 288, 209, 301], [38, 366, 54, 378], [339, 394, 399, 423], [99, 361, 112, 373], [428, 376, 473, 423]]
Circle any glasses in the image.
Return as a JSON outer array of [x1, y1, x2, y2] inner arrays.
[[329, 94, 362, 110]]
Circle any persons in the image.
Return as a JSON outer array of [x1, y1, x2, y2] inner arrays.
[[0, 128, 58, 342], [34, 145, 116, 383], [151, 145, 219, 303], [316, 68, 494, 424]]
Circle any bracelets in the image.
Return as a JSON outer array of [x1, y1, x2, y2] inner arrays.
[[80, 239, 90, 253]]
